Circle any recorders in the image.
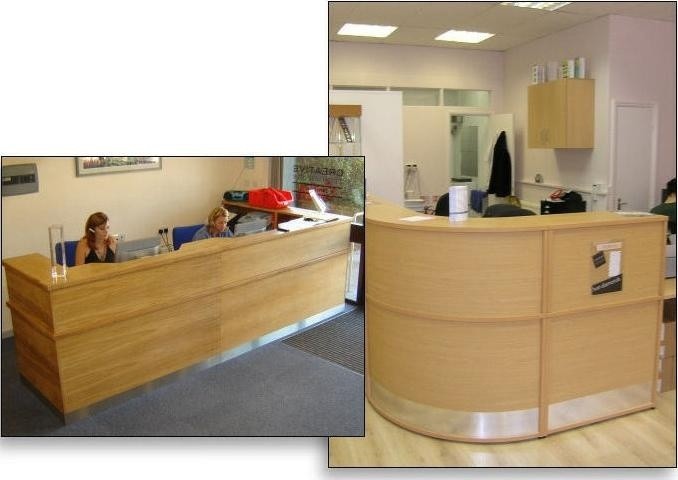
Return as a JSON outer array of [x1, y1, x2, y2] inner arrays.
[[223, 191, 249, 201]]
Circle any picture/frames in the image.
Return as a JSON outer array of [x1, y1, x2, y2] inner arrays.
[[77, 156, 162, 175]]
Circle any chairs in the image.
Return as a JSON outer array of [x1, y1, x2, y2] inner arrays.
[[56, 240, 82, 267], [172, 224, 208, 250]]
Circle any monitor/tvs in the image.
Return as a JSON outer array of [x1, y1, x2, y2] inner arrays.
[[114, 236, 160, 263], [234, 220, 267, 237]]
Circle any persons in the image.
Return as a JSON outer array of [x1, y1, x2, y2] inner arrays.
[[646, 177, 676, 244], [191, 207, 234, 240], [74, 210, 118, 265]]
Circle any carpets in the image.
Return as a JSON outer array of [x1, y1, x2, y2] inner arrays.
[[281, 305, 363, 379]]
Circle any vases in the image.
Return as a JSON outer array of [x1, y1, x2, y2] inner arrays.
[[48, 224, 65, 281]]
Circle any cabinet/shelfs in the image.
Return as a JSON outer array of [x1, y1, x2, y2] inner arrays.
[[524, 77, 595, 150]]
